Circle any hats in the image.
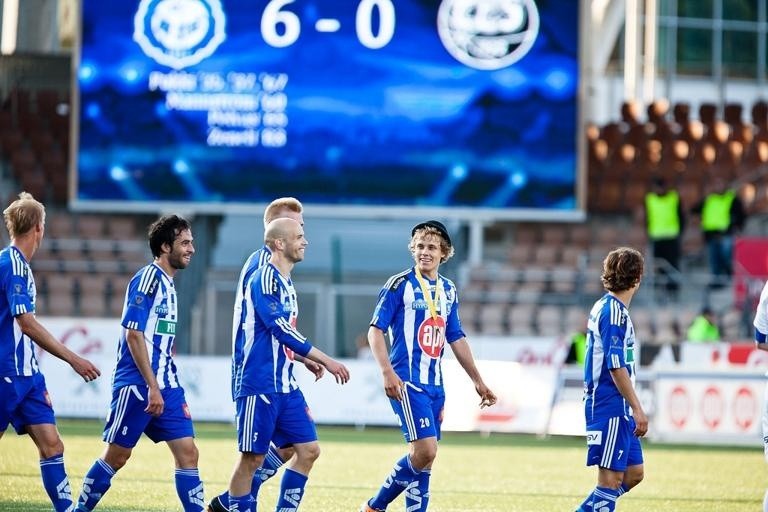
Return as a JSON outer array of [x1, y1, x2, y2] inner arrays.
[[411, 219, 452, 250]]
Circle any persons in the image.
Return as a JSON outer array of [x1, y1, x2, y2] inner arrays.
[[77, 212, 207, 512], [354, 219, 500, 511], [226, 218, 352, 512], [642, 175, 692, 299], [1, 190, 102, 512], [686, 304, 722, 343], [208, 196, 326, 512], [752, 277, 768, 512], [687, 176, 749, 293], [564, 321, 589, 364], [574, 247, 653, 512]]
